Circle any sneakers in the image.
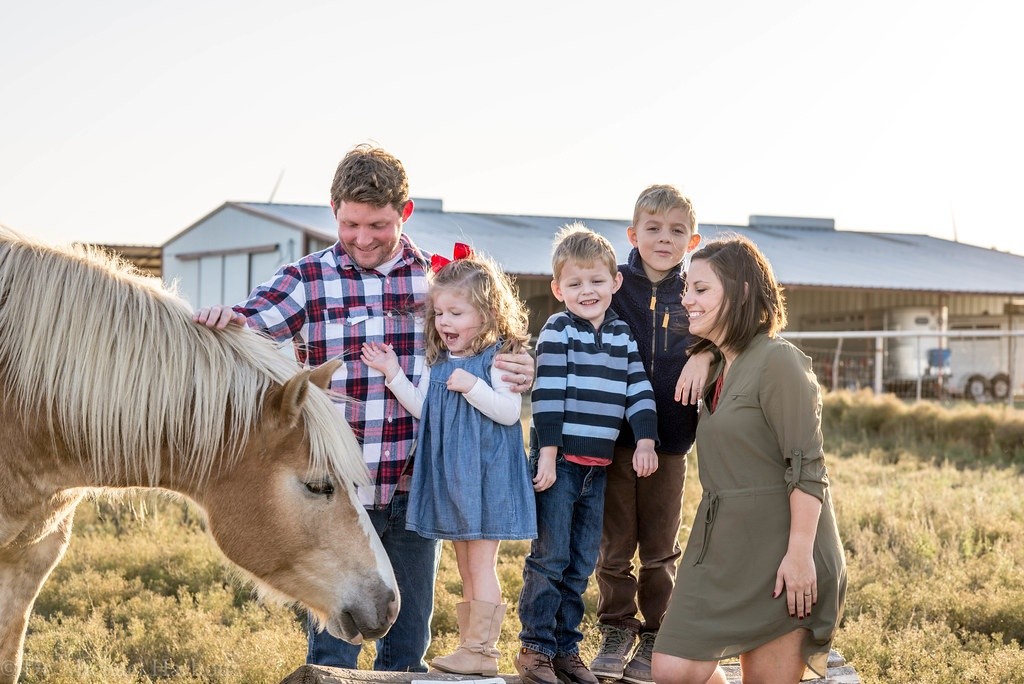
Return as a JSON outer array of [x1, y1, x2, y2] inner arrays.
[[591, 620, 656, 684], [515, 646, 601, 684]]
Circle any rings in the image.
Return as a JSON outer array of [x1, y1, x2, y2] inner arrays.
[[805, 593, 812, 597], [523, 375, 527, 385]]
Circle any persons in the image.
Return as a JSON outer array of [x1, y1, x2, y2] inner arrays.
[[512, 223, 660, 684], [651, 239, 847, 684], [191, 142, 534, 673], [587, 184, 725, 684], [360, 243, 539, 677]]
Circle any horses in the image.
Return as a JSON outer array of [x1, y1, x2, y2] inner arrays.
[[1, 222, 402, 684]]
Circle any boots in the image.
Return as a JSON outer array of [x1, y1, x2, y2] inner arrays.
[[428, 601, 508, 676]]
